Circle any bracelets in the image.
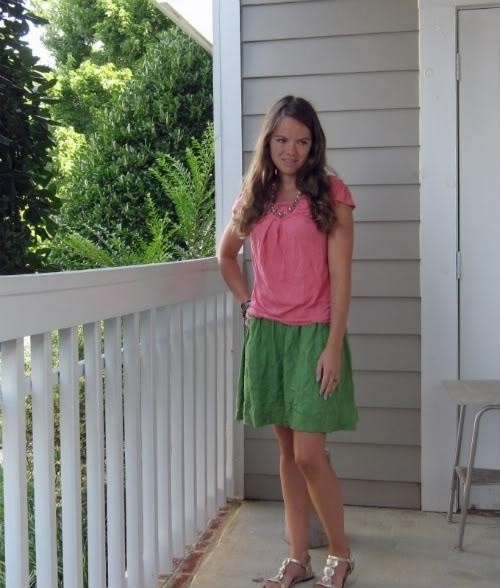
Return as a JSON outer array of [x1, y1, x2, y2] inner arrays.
[[240, 298, 251, 318]]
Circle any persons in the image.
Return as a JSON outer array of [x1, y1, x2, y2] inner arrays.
[[217, 94, 354, 588]]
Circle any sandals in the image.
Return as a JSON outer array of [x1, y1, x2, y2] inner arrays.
[[263, 548, 355, 588]]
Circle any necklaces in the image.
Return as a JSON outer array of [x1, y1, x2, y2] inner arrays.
[[269, 180, 303, 218]]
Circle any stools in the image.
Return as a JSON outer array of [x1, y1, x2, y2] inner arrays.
[[441, 379, 500, 552]]
